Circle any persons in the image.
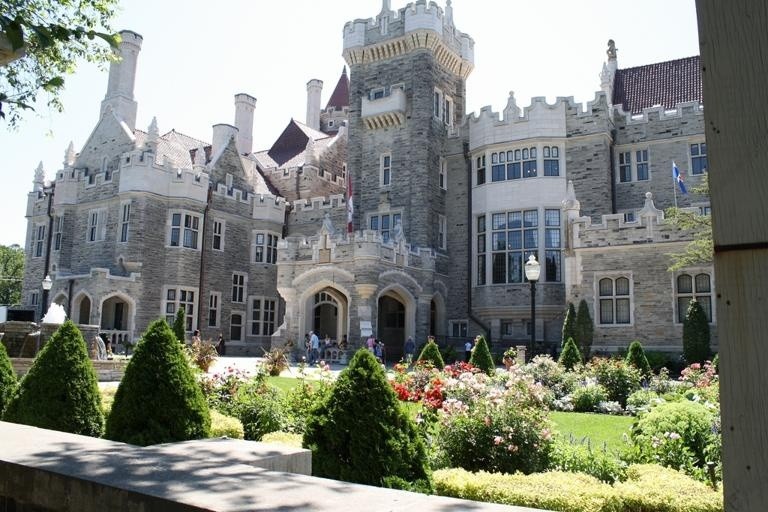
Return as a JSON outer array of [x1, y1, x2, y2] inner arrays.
[[464, 338, 473, 363], [302, 329, 387, 367], [403, 334, 417, 362], [191, 329, 201, 350], [217, 332, 226, 358]]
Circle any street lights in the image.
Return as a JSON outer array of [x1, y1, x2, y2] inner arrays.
[[525, 254, 540, 361], [39, 275, 52, 351]]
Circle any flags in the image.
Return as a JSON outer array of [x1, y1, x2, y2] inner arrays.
[[672, 161, 688, 195], [346, 172, 355, 231]]
